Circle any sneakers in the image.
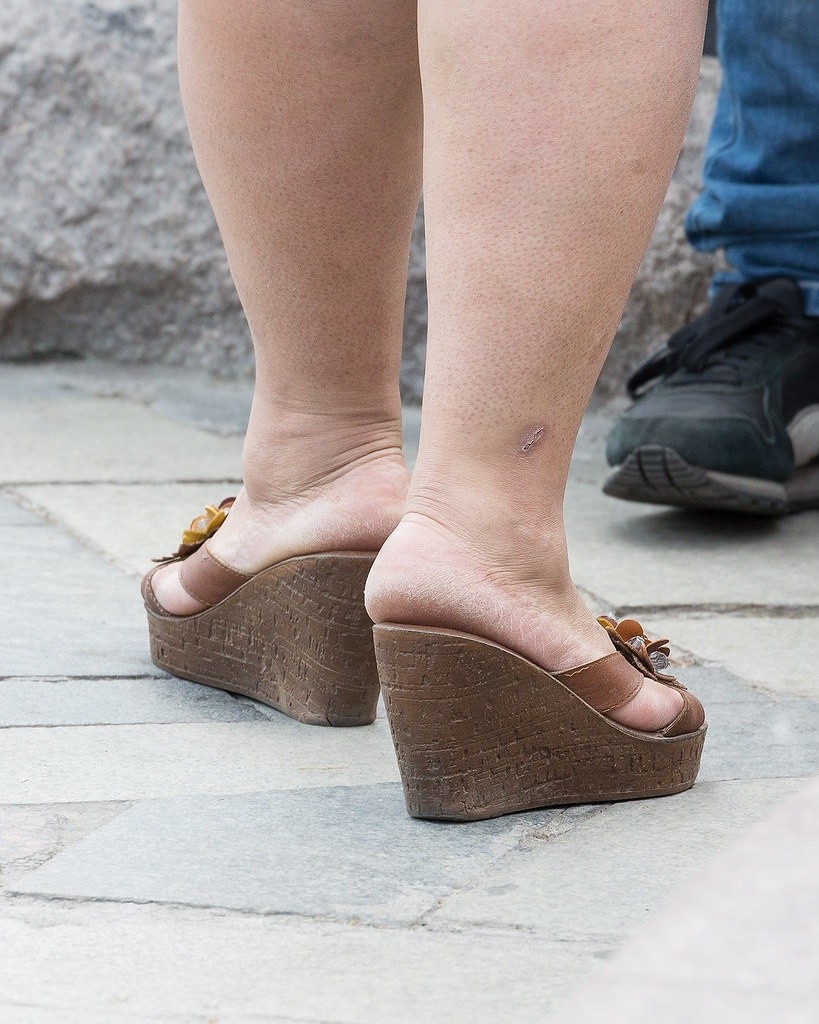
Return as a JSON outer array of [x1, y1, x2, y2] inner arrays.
[[602, 276, 819, 516]]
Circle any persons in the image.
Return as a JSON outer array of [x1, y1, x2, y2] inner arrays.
[[602, 0, 819, 514], [140, 0, 712, 822]]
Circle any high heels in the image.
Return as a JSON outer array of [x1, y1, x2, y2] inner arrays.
[[373, 609, 707, 824], [139, 496, 381, 728]]
